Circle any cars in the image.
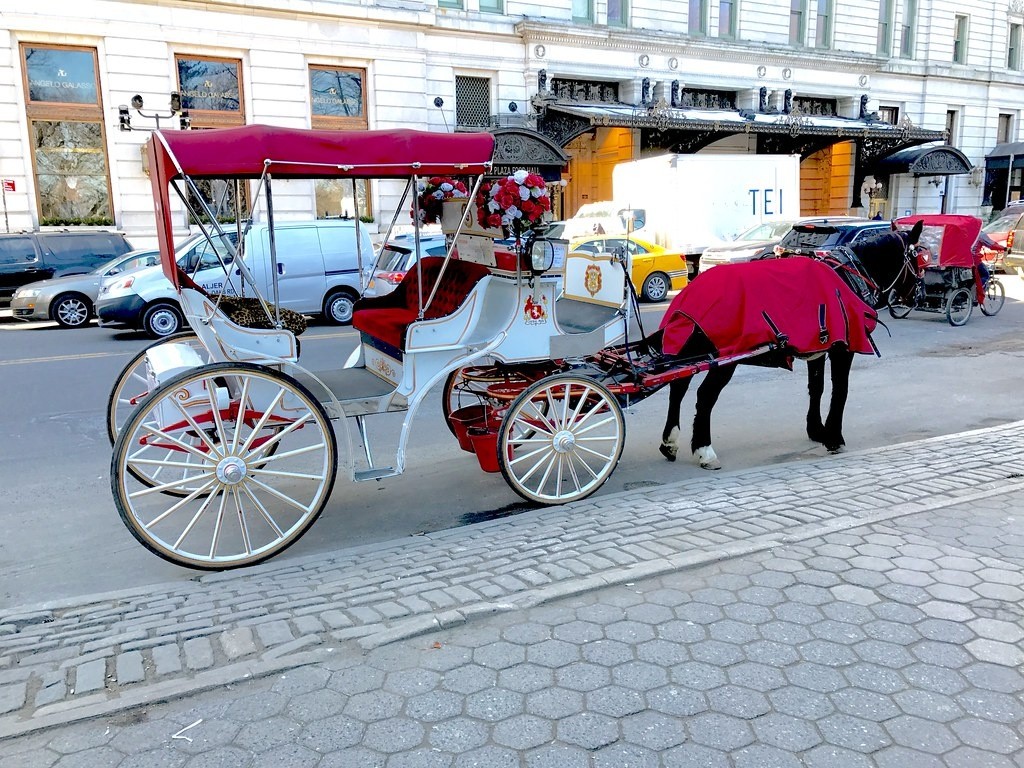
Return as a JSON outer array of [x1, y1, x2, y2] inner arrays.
[[361, 229, 545, 301], [519, 221, 565, 244], [10, 246, 228, 329], [569, 234, 689, 303], [974, 199, 1024, 274]]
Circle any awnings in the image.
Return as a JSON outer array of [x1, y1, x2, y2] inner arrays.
[[539, 102, 949, 208], [985, 141, 1024, 208], [875, 145, 975, 217]]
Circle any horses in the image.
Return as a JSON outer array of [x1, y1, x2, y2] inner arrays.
[[636, 218, 926, 469]]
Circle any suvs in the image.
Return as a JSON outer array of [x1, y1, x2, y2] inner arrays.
[[0, 228, 140, 308], [698, 215, 893, 306]]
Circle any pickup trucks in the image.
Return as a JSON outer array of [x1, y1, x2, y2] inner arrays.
[[93, 219, 376, 339]]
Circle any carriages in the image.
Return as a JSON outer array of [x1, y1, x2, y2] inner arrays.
[[104, 125, 926, 573]]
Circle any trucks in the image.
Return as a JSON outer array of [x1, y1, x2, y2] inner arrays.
[[555, 152, 802, 286]]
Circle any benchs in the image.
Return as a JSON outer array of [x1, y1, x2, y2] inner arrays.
[[440, 198, 568, 284], [175, 264, 307, 367], [351, 255, 492, 355]]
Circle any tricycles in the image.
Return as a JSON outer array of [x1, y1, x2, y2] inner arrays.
[[886, 213, 1012, 326]]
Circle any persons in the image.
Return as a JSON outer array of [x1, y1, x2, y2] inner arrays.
[[971, 230, 1012, 307], [871, 211, 883, 220]]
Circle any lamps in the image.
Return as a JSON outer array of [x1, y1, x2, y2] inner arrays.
[[929, 175, 942, 188], [740, 108, 755, 121], [521, 218, 555, 303], [864, 113, 875, 127], [969, 165, 983, 190]]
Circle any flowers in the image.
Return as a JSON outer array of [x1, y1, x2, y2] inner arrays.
[[410, 176, 470, 226], [478, 172, 552, 238]]
